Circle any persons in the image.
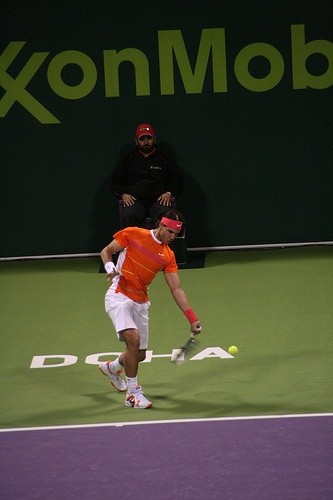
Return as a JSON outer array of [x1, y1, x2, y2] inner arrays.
[[97, 213, 202, 410], [111, 123, 191, 268]]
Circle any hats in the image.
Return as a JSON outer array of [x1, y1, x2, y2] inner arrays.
[[135, 123, 155, 141]]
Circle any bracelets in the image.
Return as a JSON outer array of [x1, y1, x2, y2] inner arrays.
[[166, 192, 171, 195], [105, 261, 117, 273], [184, 307, 199, 324]]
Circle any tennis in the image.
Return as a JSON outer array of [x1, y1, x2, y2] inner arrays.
[[229, 345, 238, 355]]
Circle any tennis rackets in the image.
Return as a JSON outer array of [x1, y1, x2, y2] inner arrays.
[[170, 324, 201, 367]]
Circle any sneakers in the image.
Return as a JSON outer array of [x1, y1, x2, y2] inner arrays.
[[125, 386, 152, 409], [99, 360, 127, 392]]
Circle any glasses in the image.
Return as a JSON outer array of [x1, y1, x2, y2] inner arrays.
[[138, 136, 153, 141]]
